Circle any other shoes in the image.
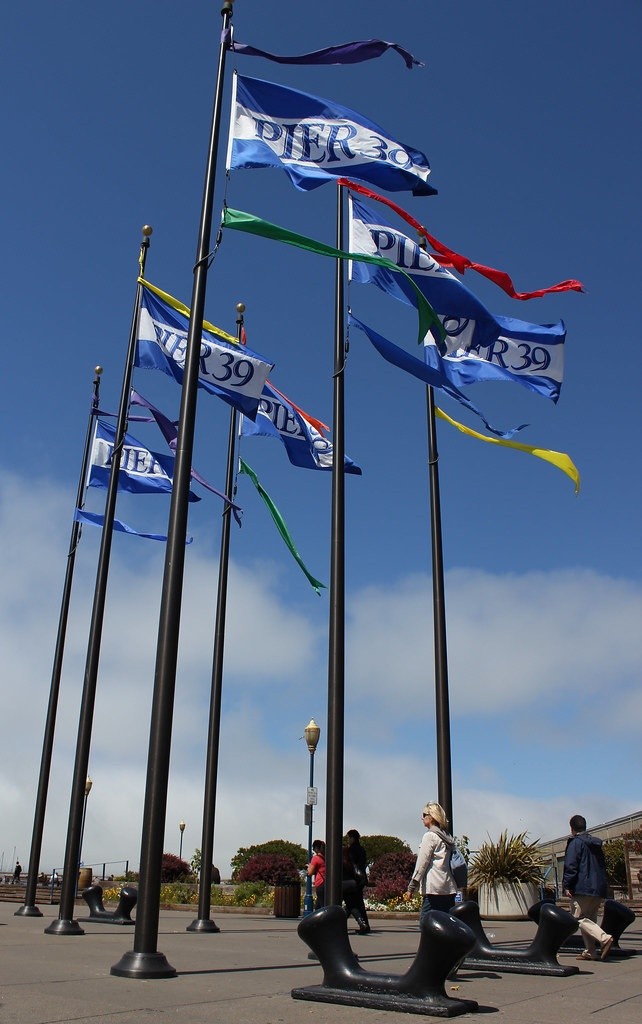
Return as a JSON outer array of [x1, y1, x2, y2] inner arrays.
[[447, 956, 466, 979], [600, 937, 614, 959], [576, 954, 597, 960]]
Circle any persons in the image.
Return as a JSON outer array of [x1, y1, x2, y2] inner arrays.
[[51, 873, 59, 887], [12, 862, 21, 885], [342, 830, 370, 935], [403, 803, 465, 979], [307, 840, 325, 909], [562, 815, 612, 959], [108, 875, 113, 881]]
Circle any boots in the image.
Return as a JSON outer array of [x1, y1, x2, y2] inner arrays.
[[356, 915, 368, 935]]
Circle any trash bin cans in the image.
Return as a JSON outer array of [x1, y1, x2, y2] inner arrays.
[[274, 880, 301, 918]]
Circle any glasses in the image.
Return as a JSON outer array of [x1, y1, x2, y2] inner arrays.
[[423, 813, 431, 818]]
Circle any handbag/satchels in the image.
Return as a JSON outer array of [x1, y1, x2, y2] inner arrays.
[[342, 869, 359, 888], [450, 848, 468, 887]]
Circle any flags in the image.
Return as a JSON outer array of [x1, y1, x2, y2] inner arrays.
[[133, 287, 275, 422], [424, 314, 567, 405], [348, 194, 501, 350], [238, 384, 363, 475], [87, 419, 202, 502], [228, 70, 439, 197]]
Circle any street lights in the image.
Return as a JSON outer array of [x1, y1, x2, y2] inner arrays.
[[178, 821, 186, 860], [75, 775, 93, 899], [301, 716, 321, 916]]
